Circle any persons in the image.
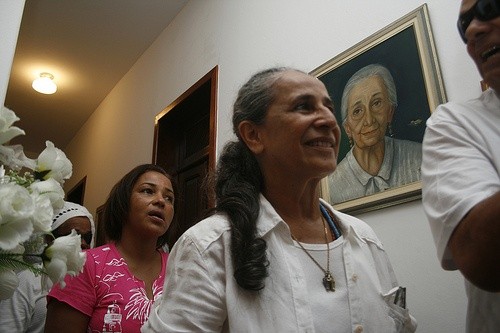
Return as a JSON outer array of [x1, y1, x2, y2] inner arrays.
[[421, 0, 500, 333], [0, 201, 95, 333], [139, 67, 417, 333], [44, 164, 180, 333]]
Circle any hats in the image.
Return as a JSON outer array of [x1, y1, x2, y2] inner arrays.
[[50, 200, 95, 249]]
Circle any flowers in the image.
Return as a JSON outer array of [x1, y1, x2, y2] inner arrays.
[[0, 105, 87, 301]]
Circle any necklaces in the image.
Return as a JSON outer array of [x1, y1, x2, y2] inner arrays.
[[292, 214, 336, 293]]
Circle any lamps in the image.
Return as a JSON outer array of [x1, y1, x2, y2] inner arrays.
[[33, 73, 57, 95]]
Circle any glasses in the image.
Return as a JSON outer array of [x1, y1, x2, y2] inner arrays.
[[54, 231, 92, 244], [457, 0, 500, 44]]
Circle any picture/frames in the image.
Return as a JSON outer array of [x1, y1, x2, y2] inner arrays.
[[307, 3, 451, 216]]
